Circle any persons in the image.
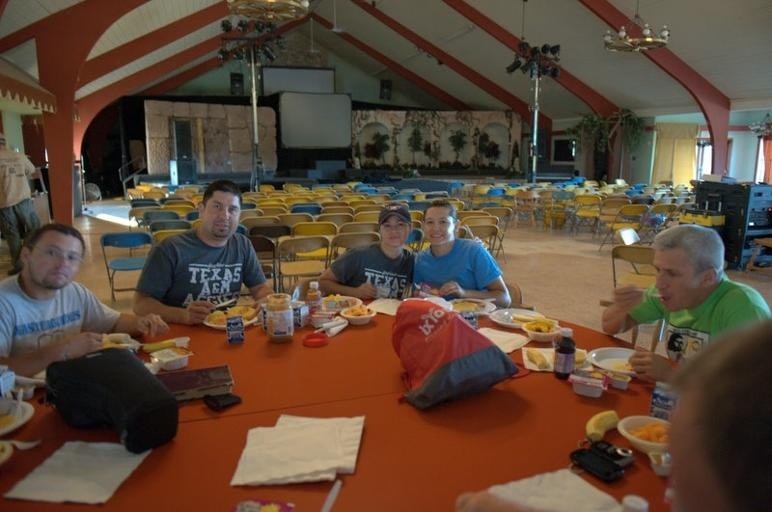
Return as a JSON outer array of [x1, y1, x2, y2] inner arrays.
[[0, 223, 170, 377], [456, 318, 772, 512], [410, 201, 512, 308], [317, 202, 415, 301], [0, 133, 43, 276], [131, 180, 275, 325], [601, 224, 772, 384]]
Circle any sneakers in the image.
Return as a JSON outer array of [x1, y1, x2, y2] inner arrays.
[[8, 266, 22, 276]]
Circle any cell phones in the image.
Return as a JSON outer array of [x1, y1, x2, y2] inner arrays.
[[570, 447, 624, 483]]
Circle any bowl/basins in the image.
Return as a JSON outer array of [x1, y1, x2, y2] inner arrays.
[[341, 307, 377, 326], [522, 322, 562, 342]]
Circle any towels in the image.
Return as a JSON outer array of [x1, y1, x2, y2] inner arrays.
[[475, 327, 531, 353], [322, 316, 350, 338], [274, 415, 365, 476], [523, 346, 595, 374], [365, 298, 402, 315], [2, 441, 152, 504], [493, 467, 624, 511], [229, 423, 345, 490]]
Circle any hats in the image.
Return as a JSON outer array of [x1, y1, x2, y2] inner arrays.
[[379, 203, 412, 226]]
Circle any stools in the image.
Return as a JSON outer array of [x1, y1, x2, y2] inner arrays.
[[745, 237, 772, 278]]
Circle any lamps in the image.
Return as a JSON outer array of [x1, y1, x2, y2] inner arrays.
[[521, 44, 561, 183], [217, 18, 286, 192], [602, 1, 670, 53], [302, 16, 320, 55], [505, 0, 529, 73], [328, 0, 343, 33]]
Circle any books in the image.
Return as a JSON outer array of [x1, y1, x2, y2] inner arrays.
[[158, 365, 235, 402]]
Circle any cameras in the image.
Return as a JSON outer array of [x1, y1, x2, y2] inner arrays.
[[589, 439, 633, 467]]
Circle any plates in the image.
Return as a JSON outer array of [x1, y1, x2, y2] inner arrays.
[[447, 298, 496, 316], [586, 347, 641, 377], [617, 415, 675, 454], [0, 398, 35, 435], [489, 308, 545, 329], [203, 308, 259, 329], [319, 296, 363, 315]]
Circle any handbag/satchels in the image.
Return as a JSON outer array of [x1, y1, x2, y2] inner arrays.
[[391, 298, 522, 410]]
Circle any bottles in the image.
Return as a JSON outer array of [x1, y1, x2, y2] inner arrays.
[[553, 328, 577, 380], [266, 293, 294, 343], [308, 282, 321, 301]]
